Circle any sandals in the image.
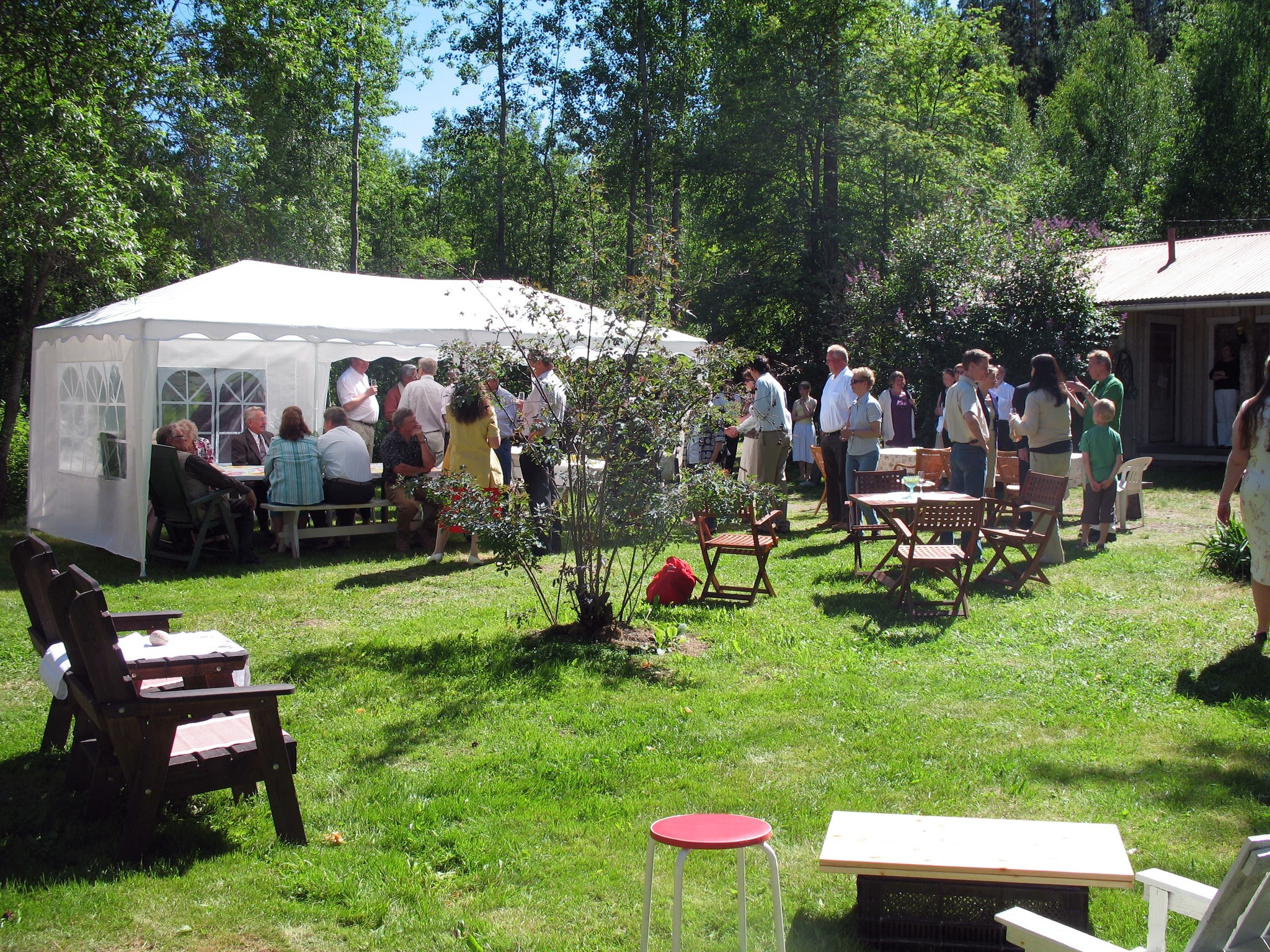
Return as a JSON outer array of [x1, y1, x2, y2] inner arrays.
[[1095, 544, 1104, 550], [1077, 543, 1087, 549]]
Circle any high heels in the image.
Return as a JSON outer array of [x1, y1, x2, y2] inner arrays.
[[469, 556, 484, 567], [427, 553, 443, 564]]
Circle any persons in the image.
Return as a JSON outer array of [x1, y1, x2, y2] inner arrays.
[[790, 381, 817, 481], [609, 353, 791, 534], [335, 358, 380, 517], [800, 345, 1125, 564], [1218, 356, 1270, 643], [231, 407, 274, 547], [146, 419, 266, 563], [264, 407, 326, 550], [308, 407, 376, 547], [384, 351, 566, 565], [1209, 345, 1239, 450]]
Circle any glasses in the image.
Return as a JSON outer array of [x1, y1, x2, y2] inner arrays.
[[955, 371, 964, 374], [851, 379, 865, 384], [174, 435, 185, 439], [405, 374, 418, 379], [251, 417, 266, 422]]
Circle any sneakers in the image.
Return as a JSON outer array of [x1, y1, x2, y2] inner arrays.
[[800, 480, 816, 486], [395, 533, 410, 552], [413, 531, 436, 549]]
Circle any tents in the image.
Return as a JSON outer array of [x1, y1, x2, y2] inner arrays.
[[28, 260, 709, 579]]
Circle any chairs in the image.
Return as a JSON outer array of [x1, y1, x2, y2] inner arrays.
[[811, 445, 1153, 618], [11, 533, 251, 795], [47, 564, 308, 864], [995, 832, 1270, 952], [692, 495, 784, 607], [148, 444, 242, 569]]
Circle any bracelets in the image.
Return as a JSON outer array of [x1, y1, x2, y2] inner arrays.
[[1219, 502, 1229, 505]]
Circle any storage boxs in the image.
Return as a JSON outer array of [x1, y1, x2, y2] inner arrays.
[[857, 875, 1090, 952]]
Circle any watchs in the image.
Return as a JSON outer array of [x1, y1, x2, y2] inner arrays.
[[418, 438, 427, 443], [1083, 390, 1091, 396], [852, 430, 854, 436]]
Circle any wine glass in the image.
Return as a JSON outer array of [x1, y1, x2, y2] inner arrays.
[[903, 475, 920, 499], [917, 471, 925, 498], [840, 421, 847, 440]]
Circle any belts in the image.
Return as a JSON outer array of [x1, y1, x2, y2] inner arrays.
[[426, 430, 440, 433], [359, 421, 373, 427]]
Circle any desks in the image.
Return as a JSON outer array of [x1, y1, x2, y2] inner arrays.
[[876, 447, 939, 474], [848, 491, 978, 604], [222, 462, 442, 524], [1059, 453, 1086, 522]]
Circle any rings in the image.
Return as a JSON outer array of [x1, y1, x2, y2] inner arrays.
[[842, 438, 844, 439]]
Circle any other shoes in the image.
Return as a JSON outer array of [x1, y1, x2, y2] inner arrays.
[[773, 527, 789, 533], [1039, 557, 1048, 563], [1251, 631, 1268, 643], [816, 519, 881, 542], [1019, 520, 1032, 529], [746, 528, 770, 535], [1078, 528, 1116, 542], [271, 536, 352, 554], [238, 554, 266, 565], [977, 557, 987, 564]]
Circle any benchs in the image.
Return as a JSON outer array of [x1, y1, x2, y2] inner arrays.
[[261, 499, 425, 559]]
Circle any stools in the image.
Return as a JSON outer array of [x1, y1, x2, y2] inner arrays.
[[640, 814, 785, 952]]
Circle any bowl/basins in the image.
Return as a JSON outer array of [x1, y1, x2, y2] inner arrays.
[[908, 446, 924, 452]]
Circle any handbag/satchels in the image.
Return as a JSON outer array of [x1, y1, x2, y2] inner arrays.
[[647, 556, 703, 605]]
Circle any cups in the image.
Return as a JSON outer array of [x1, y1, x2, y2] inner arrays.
[[1010, 407, 1017, 415], [518, 392, 524, 400], [371, 379, 376, 386]]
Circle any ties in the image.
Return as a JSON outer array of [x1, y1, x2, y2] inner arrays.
[[258, 435, 267, 465]]
